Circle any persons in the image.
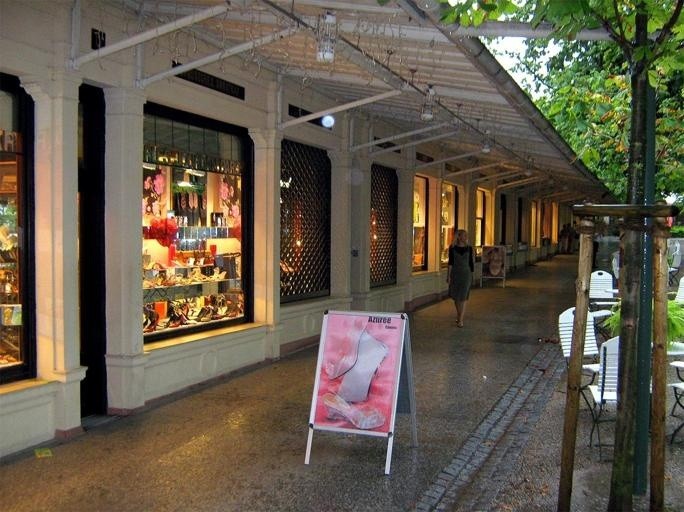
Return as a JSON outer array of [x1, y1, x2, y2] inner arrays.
[[558, 223, 569, 253], [567, 222, 576, 254], [445, 229, 475, 329]]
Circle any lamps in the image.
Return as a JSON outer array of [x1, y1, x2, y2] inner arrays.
[[314, 8, 492, 154]]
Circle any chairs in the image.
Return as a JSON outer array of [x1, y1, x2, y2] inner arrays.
[[557, 238, 684, 463]]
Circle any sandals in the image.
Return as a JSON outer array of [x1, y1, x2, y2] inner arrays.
[[455, 318, 464, 328]]
